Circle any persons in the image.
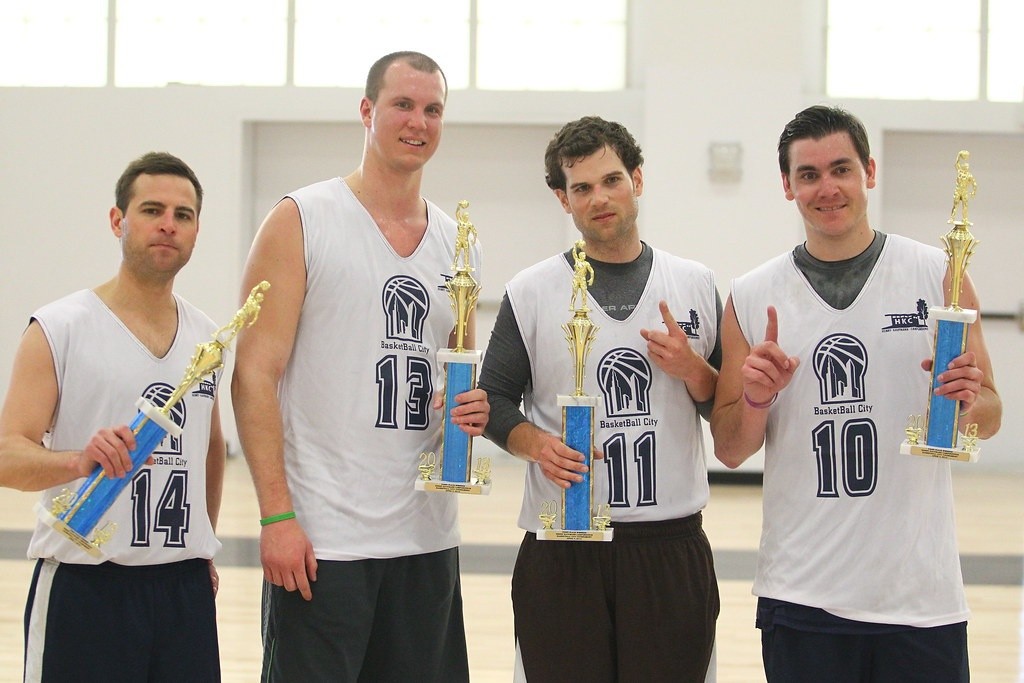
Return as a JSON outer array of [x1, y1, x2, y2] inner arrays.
[[477, 119, 728, 682], [1, 151, 226, 681], [260, 508, 297, 527], [231, 49, 493, 681], [711, 104, 1004, 683]]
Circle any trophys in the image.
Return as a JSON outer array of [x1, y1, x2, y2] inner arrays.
[[40, 278, 274, 560], [900, 145, 982, 465], [528, 237, 616, 544], [414, 199, 493, 499]]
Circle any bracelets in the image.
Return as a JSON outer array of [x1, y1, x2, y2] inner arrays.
[[743, 389, 779, 409]]
